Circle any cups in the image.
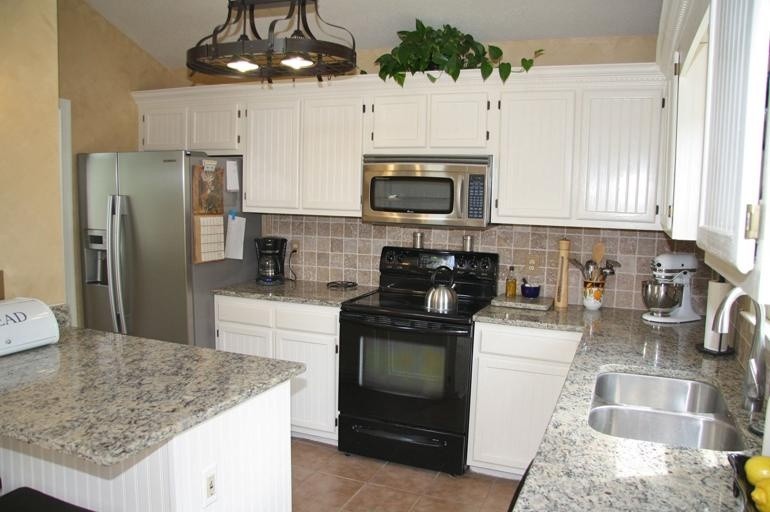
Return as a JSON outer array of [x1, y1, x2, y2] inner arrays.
[[583, 281, 604, 310]]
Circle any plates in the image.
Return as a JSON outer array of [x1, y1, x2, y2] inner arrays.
[[727, 452, 759, 512]]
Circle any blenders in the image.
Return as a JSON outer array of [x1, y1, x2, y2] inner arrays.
[[642, 251, 704, 323]]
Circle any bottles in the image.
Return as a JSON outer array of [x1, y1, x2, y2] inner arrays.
[[505, 266, 516, 297], [413, 232, 423, 249], [464, 235, 473, 253], [554, 236, 569, 312]]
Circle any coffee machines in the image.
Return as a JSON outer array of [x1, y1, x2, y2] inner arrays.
[[254, 236, 287, 284]]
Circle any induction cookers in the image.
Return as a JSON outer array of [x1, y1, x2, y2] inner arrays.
[[341, 290, 489, 324]]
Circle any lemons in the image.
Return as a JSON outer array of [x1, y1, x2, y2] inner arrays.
[[750, 478, 770, 512], [744, 455, 770, 486]]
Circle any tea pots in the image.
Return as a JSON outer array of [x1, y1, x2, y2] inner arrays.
[[423, 266, 460, 314]]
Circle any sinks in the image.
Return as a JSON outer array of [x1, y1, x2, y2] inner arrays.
[[595, 363, 728, 416], [588, 404, 748, 453]]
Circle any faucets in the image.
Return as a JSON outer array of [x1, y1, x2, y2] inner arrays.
[[711, 286, 768, 412]]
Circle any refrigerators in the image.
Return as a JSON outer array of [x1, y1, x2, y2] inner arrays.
[[74, 151, 263, 349]]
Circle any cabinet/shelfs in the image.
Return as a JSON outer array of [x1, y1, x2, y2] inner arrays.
[[490, 88, 667, 232], [241, 96, 367, 219], [213, 294, 341, 447], [467, 321, 583, 481], [141, 102, 243, 158], [363, 91, 492, 157]]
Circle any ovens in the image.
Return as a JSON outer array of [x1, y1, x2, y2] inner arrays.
[[337, 312, 473, 477]]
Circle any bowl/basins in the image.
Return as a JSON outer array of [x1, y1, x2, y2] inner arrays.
[[521, 285, 541, 298]]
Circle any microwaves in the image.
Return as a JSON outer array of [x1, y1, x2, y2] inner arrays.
[[361, 155, 490, 230]]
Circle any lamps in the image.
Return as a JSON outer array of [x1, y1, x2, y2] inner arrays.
[[185, 0, 356, 84]]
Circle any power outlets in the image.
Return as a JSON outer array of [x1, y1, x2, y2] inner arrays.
[[293, 244, 298, 254]]
